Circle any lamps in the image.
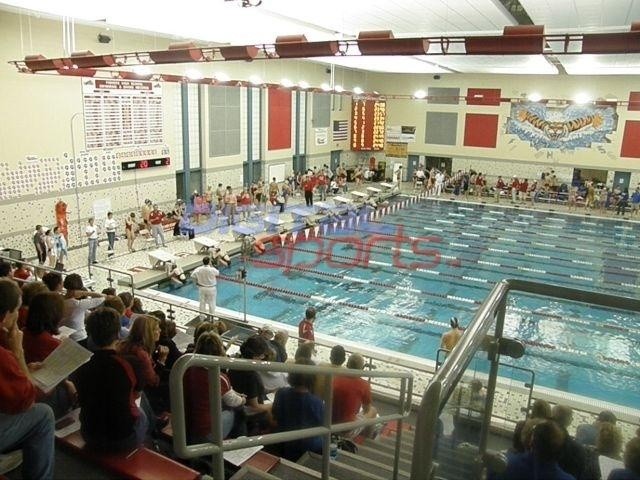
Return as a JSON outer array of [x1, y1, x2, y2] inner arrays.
[[132, 63, 593, 106]]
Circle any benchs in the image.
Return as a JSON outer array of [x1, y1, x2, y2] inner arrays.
[[482, 191, 640, 212], [54, 412, 412, 480], [141, 217, 178, 237]]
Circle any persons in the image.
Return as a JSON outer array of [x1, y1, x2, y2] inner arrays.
[[395, 163, 639, 218], [33, 162, 378, 269], [438, 318, 639, 480], [1, 257, 378, 480]]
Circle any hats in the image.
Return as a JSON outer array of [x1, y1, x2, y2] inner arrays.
[[450, 317, 459, 327]]
